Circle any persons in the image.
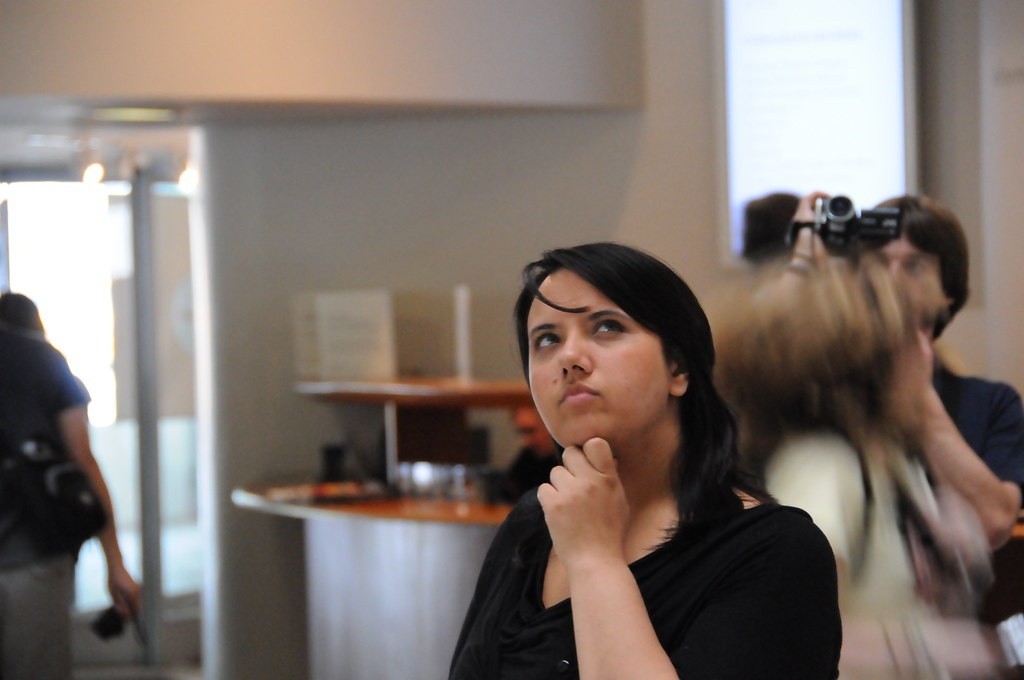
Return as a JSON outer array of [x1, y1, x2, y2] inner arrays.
[[448, 241, 844, 680], [0, 294, 144, 680], [716, 192, 1024, 680]]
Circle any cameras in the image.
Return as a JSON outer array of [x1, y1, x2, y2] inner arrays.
[[90, 605, 127, 641], [815, 196, 902, 256]]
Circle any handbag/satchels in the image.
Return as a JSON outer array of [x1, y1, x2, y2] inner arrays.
[[0, 431, 108, 556]]
